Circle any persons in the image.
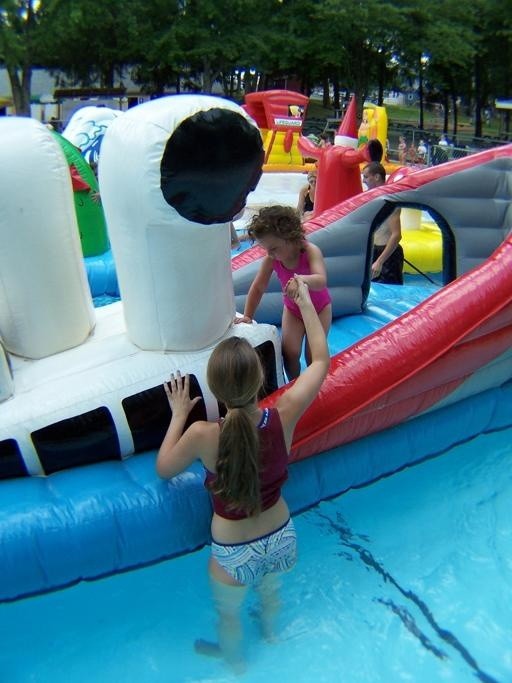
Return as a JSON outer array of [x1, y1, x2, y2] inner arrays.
[[397, 135, 408, 166], [363, 158, 405, 286], [295, 171, 318, 216], [409, 142, 417, 165], [437, 134, 449, 153], [416, 139, 427, 161], [232, 203, 334, 383], [155, 274, 330, 669]]
